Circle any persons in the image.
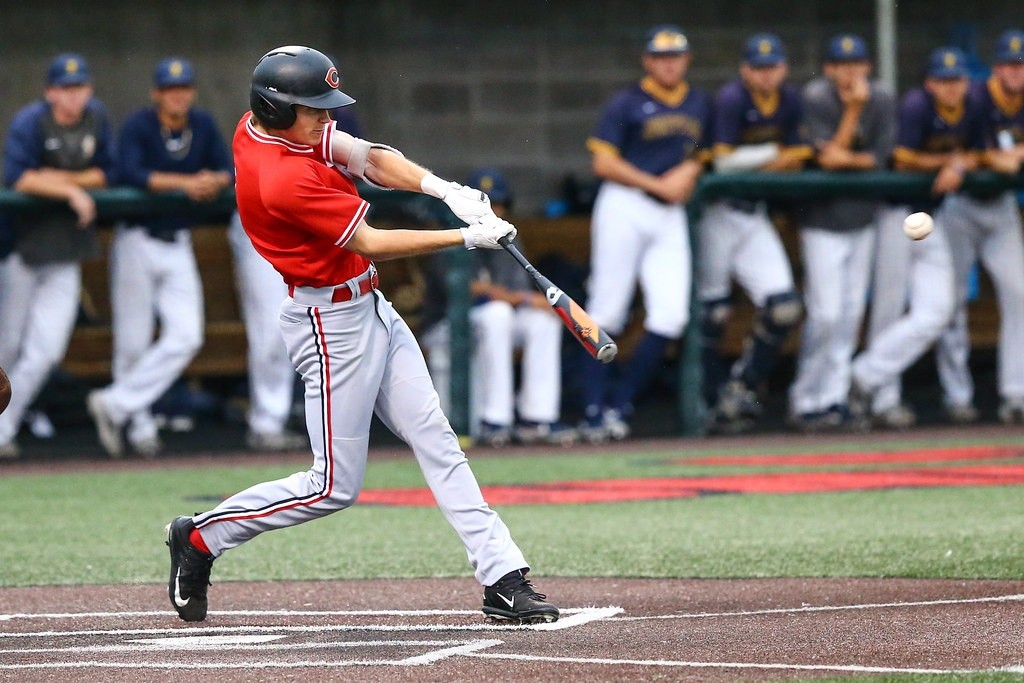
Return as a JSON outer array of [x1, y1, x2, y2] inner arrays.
[[693, 32, 805, 431], [0, 53, 115, 456], [380, 202, 449, 423], [86, 56, 235, 458], [165, 45, 561, 621], [568, 23, 713, 443], [225, 58, 341, 449], [451, 166, 560, 444], [804, 36, 899, 427], [935, 29, 1024, 426], [844, 46, 977, 436]]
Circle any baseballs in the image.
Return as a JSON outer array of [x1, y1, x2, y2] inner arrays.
[[903, 212, 935, 241]]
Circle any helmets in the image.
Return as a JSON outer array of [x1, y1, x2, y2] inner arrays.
[[250, 46, 356, 131]]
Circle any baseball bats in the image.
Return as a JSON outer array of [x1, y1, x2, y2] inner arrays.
[[499, 234, 618, 363]]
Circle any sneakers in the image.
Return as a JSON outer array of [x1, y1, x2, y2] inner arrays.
[[482, 568, 560, 625], [164, 512, 217, 621]]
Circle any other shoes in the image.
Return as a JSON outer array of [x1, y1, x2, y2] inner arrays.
[[88, 390, 123, 459], [137, 434, 159, 459], [470, 410, 632, 443], [703, 385, 1024, 436], [248, 425, 306, 452]]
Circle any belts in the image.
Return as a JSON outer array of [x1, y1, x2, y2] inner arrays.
[[288, 272, 379, 303]]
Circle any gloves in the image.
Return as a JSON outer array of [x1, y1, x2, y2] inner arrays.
[[460, 217, 517, 250], [443, 180, 492, 225]]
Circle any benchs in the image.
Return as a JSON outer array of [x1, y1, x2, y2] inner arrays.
[[0, 212, 1004, 392]]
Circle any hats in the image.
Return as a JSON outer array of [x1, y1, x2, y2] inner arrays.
[[742, 35, 788, 70], [645, 26, 687, 55], [996, 31, 1024, 63], [154, 58, 194, 88], [47, 54, 90, 88], [930, 48, 967, 80], [465, 171, 509, 207], [831, 35, 867, 61]]
